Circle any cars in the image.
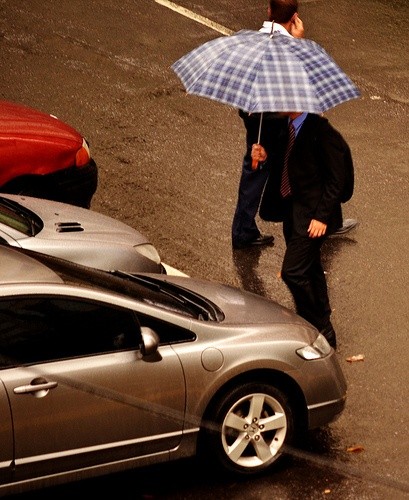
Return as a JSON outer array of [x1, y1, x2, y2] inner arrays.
[[0, 193, 192, 279], [0, 98, 99, 204], [0, 193, 168, 275], [0, 244, 349, 500]]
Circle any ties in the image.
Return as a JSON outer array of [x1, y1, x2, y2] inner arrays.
[[280, 120, 295, 197]]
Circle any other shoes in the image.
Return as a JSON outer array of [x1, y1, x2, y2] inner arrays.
[[250, 235, 274, 244], [335, 220, 355, 234], [324, 329, 336, 349]]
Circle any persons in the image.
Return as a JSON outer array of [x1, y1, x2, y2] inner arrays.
[[252, 111, 355, 353], [232, 0, 359, 245]]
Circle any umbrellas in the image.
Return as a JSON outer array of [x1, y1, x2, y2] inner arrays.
[[170, 21, 361, 169]]
